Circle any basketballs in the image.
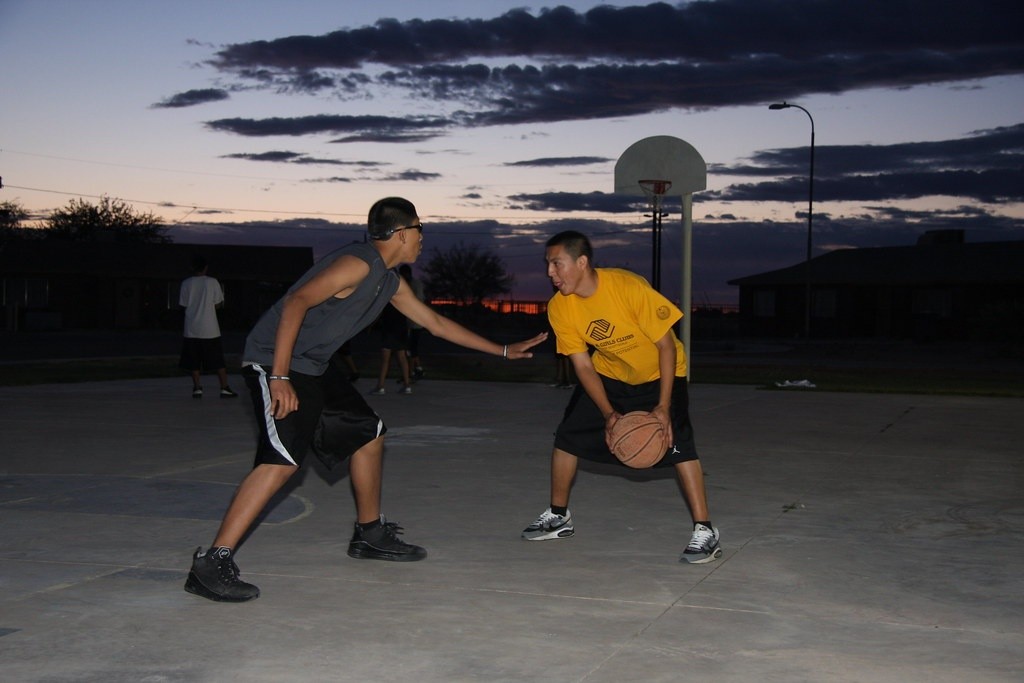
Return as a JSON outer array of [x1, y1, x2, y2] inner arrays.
[[610, 411, 669, 468]]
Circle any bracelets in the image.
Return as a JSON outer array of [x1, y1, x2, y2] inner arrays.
[[269, 375, 290, 380], [504, 344, 507, 358]]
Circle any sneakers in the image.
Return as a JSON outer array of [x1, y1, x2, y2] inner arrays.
[[368, 386, 384, 395], [192, 386, 203, 398], [221, 386, 238, 398], [680, 524, 722, 563], [399, 385, 412, 394], [522, 507, 574, 540], [184, 548, 260, 601], [349, 520, 428, 562]]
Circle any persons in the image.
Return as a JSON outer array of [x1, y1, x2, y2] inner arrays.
[[178, 257, 238, 398], [327, 264, 424, 395], [167, 196, 548, 602], [549, 285, 574, 389], [520, 230, 722, 564]]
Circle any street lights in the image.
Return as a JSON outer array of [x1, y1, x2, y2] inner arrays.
[[768, 102, 814, 340]]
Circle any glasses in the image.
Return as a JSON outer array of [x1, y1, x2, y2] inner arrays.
[[385, 222, 423, 234]]
